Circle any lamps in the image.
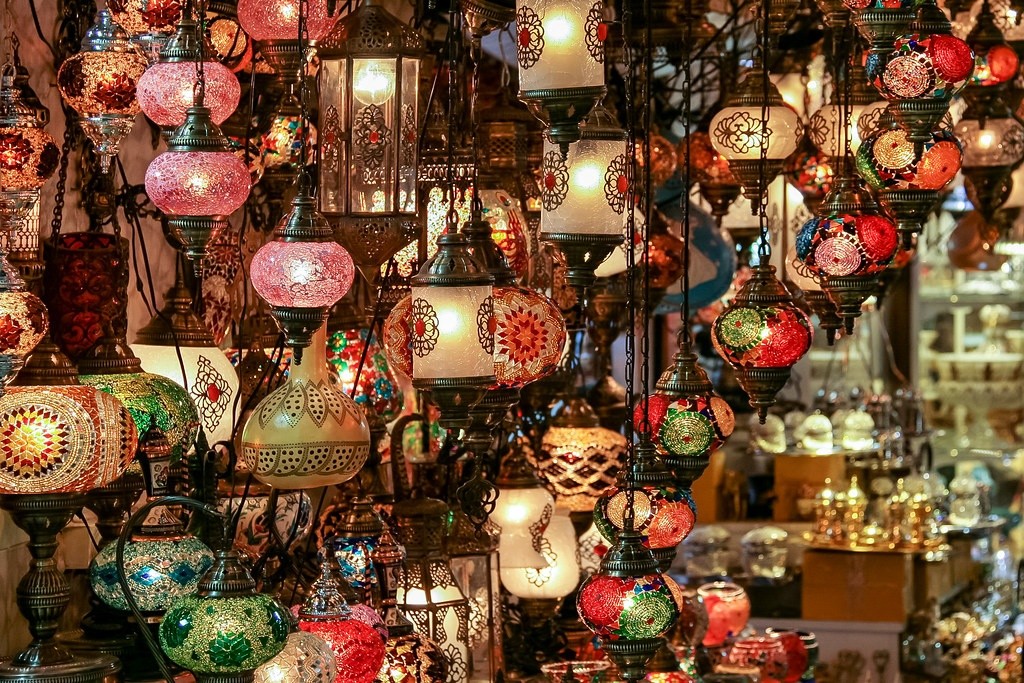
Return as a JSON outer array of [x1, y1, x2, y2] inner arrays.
[[0, 0, 1024, 683]]
